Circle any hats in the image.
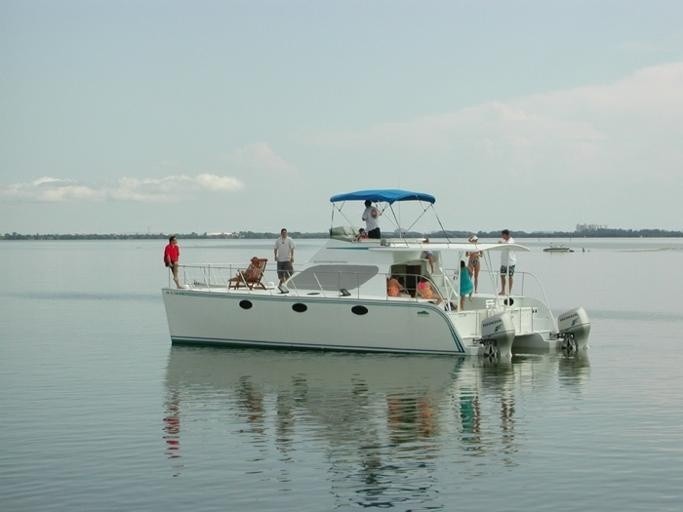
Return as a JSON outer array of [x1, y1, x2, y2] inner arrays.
[[471, 236, 478, 241]]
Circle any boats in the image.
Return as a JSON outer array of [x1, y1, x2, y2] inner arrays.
[[157, 343, 585, 462], [542, 247, 569, 252], [158, 188, 592, 368]]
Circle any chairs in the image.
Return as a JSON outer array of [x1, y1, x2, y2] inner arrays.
[[224, 258, 268, 291]]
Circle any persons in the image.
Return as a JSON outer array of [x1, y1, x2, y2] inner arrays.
[[387, 275, 408, 297], [273, 228, 295, 284], [496, 229, 516, 296], [416, 275, 441, 305], [355, 228, 367, 241], [237, 257, 262, 281], [465, 234, 483, 294], [422, 239, 434, 273], [460, 260, 473, 311], [362, 200, 382, 239], [164, 236, 184, 289]]
